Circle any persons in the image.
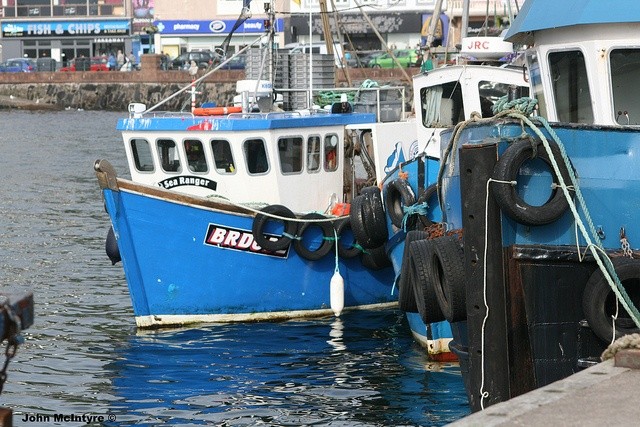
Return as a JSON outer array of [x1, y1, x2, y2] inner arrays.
[[61, 48, 200, 71]]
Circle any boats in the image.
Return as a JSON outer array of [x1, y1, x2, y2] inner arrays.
[[437, 0, 640, 413], [373, 0, 530, 361], [95, 1, 412, 334]]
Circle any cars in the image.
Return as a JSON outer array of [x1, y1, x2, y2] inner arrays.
[[59, 56, 109, 70], [359, 51, 383, 67], [220, 53, 245, 68], [1, 57, 37, 72], [367, 48, 417, 67]]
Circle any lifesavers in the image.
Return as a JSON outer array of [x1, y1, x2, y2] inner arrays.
[[193, 107, 242, 115]]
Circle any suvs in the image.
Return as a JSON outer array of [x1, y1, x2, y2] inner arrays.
[[168, 50, 219, 69]]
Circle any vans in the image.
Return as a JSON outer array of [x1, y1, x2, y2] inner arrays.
[[342, 50, 368, 67], [281, 41, 349, 67]]
